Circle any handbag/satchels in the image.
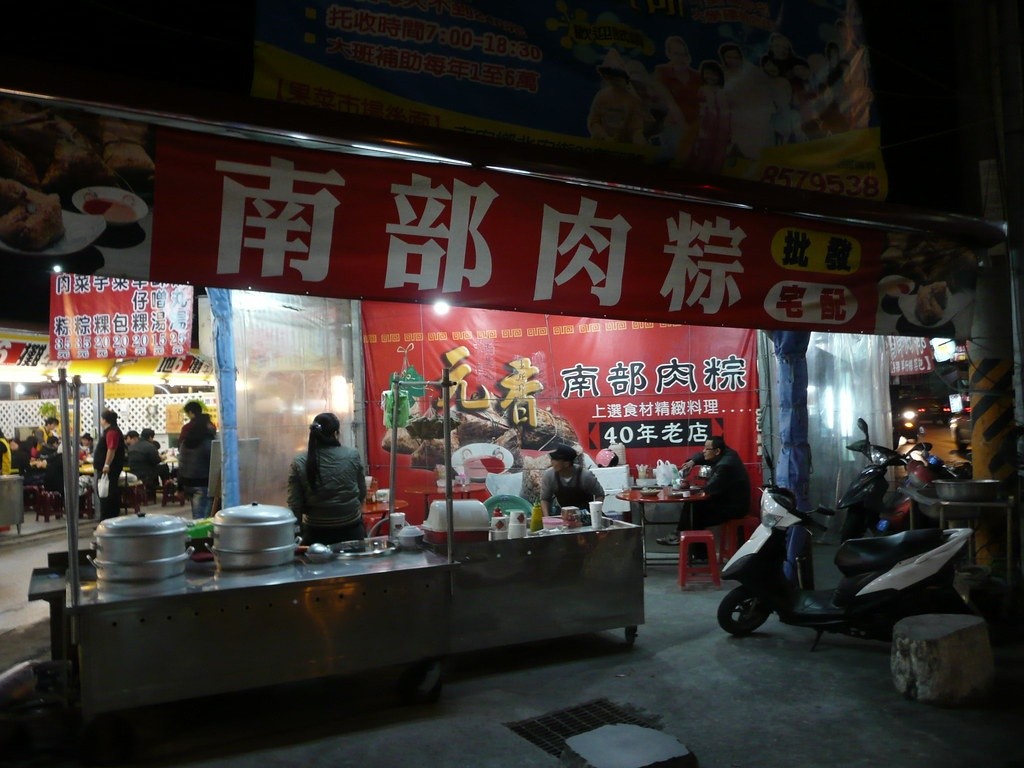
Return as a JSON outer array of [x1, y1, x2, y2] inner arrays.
[[96, 473, 109, 498]]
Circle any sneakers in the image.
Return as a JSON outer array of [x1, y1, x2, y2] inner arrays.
[[657, 534, 680, 545], [688, 555, 709, 565]]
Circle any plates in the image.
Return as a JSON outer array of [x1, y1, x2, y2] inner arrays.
[[879, 275, 964, 328], [0, 208, 107, 256], [451, 442, 514, 482], [71, 186, 147, 224], [640, 490, 661, 496]]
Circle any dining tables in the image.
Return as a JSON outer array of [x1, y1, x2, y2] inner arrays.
[[79, 464, 131, 515], [616, 488, 711, 577]]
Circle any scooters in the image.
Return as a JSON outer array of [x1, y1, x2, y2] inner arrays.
[[717, 444, 978, 652], [836, 398, 975, 546]]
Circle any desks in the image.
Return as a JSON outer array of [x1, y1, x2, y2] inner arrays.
[[359, 498, 408, 536], [404, 484, 486, 520]]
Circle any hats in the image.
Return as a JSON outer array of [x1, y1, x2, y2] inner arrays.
[[549, 445, 578, 461]]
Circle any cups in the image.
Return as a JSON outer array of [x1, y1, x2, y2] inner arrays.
[[683, 491, 690, 497], [390, 512, 405, 541], [589, 501, 603, 529]]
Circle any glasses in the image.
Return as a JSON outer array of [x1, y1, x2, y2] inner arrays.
[[704, 447, 714, 452]]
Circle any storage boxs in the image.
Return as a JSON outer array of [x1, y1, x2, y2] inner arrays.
[[589, 466, 632, 514], [420, 497, 490, 544]]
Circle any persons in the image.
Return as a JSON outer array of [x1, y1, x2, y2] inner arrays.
[[175, 402, 218, 517], [288, 414, 367, 545], [121, 425, 161, 493], [0, 428, 12, 478], [541, 446, 605, 518], [655, 436, 752, 545], [93, 412, 125, 529], [11, 418, 93, 490]]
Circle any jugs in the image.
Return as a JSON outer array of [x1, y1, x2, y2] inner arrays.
[[680, 480, 690, 489]]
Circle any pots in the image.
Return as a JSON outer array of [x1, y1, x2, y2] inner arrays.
[[205, 501, 303, 570], [86, 512, 195, 581]]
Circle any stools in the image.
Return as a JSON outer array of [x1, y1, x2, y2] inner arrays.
[[720, 516, 759, 564], [678, 531, 720, 587], [23, 478, 185, 522]]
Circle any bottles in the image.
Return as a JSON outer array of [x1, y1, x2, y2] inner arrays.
[[608, 443, 626, 464], [530, 502, 544, 532], [366, 489, 375, 503]]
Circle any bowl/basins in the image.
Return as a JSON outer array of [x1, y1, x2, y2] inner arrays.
[[636, 479, 655, 486], [931, 478, 998, 502], [490, 510, 526, 541], [396, 526, 424, 549]]
